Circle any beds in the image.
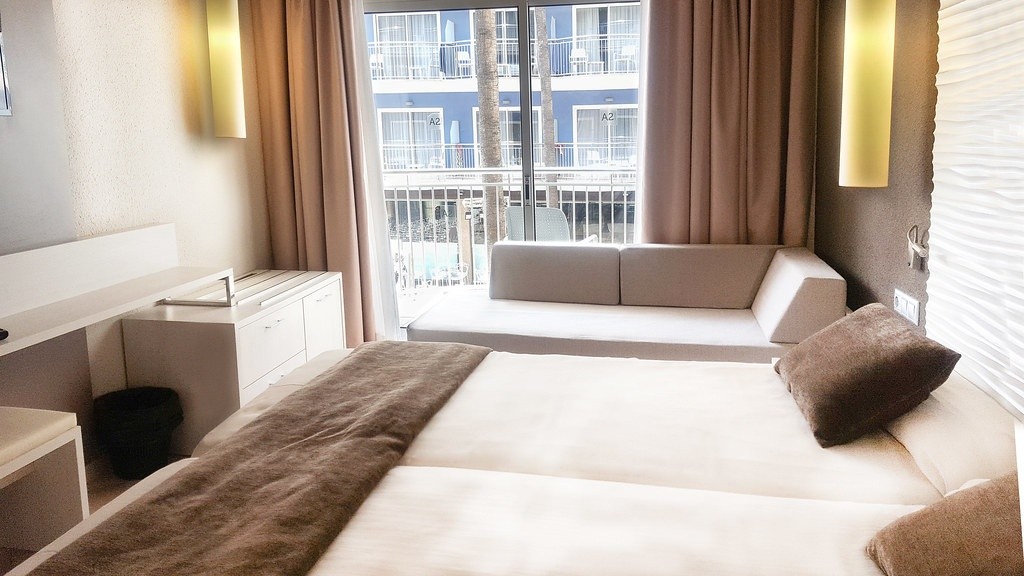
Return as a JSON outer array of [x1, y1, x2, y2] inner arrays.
[[3, 456, 1024, 576], [189, 302, 1016, 506]]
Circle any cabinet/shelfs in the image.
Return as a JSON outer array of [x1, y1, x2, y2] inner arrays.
[[121, 268, 346, 458]]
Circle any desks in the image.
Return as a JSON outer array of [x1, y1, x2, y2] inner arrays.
[[0, 267, 237, 357], [588, 61, 605, 75], [0, 406, 89, 551], [408, 164, 425, 169], [498, 64, 511, 77]]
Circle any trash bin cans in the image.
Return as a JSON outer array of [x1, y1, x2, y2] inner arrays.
[[94, 387, 183, 480]]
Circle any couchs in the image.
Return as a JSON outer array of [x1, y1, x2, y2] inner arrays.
[[406, 241, 852, 364]]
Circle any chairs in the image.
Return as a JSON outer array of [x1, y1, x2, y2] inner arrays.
[[612, 45, 638, 74], [628, 155, 637, 169], [569, 49, 588, 76], [502, 206, 599, 244], [585, 150, 604, 168], [370, 53, 386, 80], [455, 51, 473, 79]]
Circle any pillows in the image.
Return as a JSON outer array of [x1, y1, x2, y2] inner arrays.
[[863, 468, 1024, 576], [772, 303, 961, 448]]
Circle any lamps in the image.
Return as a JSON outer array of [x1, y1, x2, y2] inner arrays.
[[837, 0, 897, 187], [503, 100, 510, 105], [906, 225, 927, 269], [207, 0, 247, 139], [604, 97, 613, 102], [406, 101, 414, 105]]
